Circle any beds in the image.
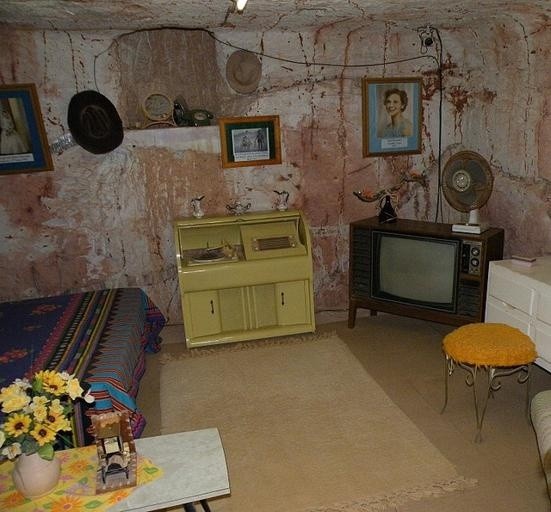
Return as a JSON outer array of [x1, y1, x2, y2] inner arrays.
[[0, 288, 166, 448]]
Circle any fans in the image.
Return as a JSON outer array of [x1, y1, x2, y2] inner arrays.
[[442, 150, 493, 235]]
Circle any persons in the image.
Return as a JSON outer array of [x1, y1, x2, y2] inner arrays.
[[380, 88, 412, 137], [242, 130, 252, 150], [255, 130, 264, 151], [1, 109, 26, 153]]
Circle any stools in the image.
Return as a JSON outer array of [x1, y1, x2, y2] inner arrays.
[[440, 322, 538, 444]]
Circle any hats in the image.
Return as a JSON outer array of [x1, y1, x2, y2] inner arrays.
[[69, 90, 122, 154], [227, 48, 261, 94]]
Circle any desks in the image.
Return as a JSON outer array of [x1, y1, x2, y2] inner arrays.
[[1, 428, 231, 511]]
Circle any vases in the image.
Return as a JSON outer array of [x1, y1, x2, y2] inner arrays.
[[12, 452, 62, 499]]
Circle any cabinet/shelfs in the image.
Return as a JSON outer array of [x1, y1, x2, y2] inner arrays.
[[171, 208, 317, 349], [484, 256, 551, 374]]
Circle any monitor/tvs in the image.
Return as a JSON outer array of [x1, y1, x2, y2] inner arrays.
[[348, 215, 505, 329]]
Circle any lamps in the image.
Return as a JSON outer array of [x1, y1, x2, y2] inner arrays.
[[236, 0, 249, 14]]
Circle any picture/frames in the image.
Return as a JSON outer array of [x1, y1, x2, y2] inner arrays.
[[219, 114, 282, 169], [361, 76, 423, 157], [0, 82, 55, 175]]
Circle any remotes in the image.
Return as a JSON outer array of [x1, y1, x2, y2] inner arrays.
[[511, 254, 536, 263]]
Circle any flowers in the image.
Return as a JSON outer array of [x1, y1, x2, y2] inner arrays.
[[0, 370, 95, 461]]
